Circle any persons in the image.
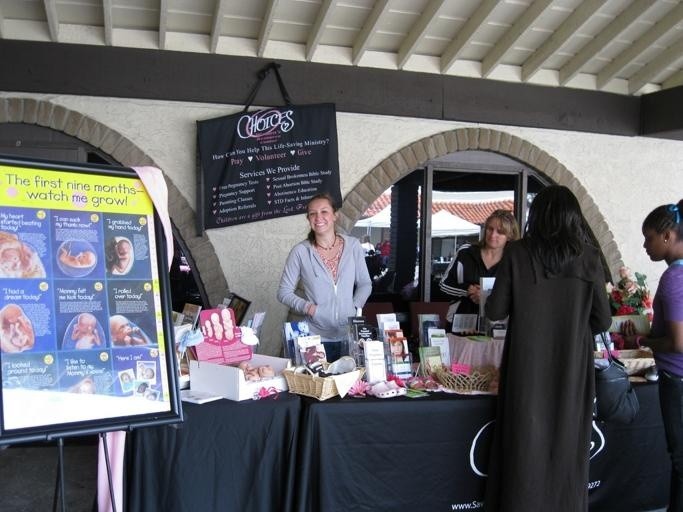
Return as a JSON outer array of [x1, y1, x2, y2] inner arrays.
[[121, 368, 157, 400], [110, 240, 131, 273], [1, 306, 32, 349], [438, 209, 520, 337], [360, 230, 390, 257], [110, 315, 149, 346], [276, 194, 373, 362], [391, 340, 408, 363], [59, 247, 96, 268], [484, 185, 612, 512], [302, 345, 325, 365], [71, 313, 101, 350], [615, 198, 683, 512]]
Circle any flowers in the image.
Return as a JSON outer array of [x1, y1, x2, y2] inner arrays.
[[605, 265, 654, 323]]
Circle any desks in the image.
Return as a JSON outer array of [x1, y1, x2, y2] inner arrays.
[[431, 260, 450, 277], [298, 366, 673, 512], [0, 387, 302, 511]]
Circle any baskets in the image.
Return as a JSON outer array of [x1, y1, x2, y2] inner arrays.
[[284, 362, 367, 402], [436, 365, 497, 392]]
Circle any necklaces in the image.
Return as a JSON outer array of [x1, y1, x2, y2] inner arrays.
[[314, 232, 336, 250]]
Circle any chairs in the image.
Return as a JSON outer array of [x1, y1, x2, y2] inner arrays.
[[362, 301, 395, 326], [409, 301, 452, 334]]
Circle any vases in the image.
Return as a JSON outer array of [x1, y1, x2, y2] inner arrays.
[[607, 314, 651, 335]]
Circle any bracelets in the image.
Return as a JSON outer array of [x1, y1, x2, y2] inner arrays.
[[636, 335, 647, 347]]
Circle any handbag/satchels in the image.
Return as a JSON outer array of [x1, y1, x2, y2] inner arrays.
[[195, 102, 342, 237], [593, 361, 641, 426]]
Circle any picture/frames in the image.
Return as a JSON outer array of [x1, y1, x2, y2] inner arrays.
[[227, 294, 251, 326], [0, 154, 185, 448]]
